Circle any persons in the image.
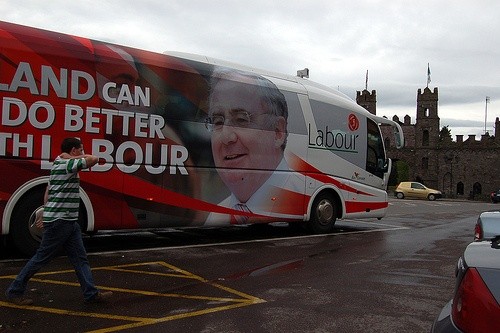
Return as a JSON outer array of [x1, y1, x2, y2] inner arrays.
[[5, 137, 113, 304], [203, 69, 307, 227]]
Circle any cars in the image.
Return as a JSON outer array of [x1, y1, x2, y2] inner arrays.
[[430, 211, 500, 333], [395, 181, 443, 201]]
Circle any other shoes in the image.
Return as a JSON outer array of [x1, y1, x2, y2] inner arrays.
[[83, 291, 113, 305], [4, 295, 33, 305]]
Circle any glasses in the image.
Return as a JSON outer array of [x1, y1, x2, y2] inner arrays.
[[205, 111, 275, 130]]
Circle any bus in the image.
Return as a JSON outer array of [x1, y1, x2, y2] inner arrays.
[[1, 21, 405, 256]]
